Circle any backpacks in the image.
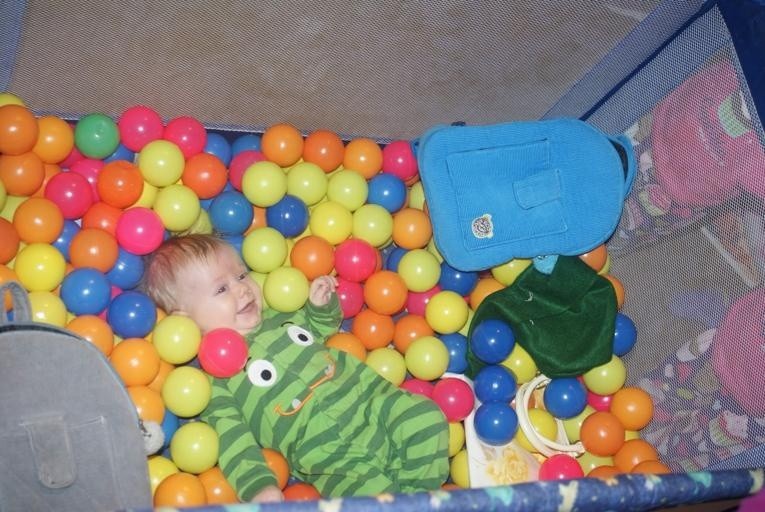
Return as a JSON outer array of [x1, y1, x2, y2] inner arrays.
[[0, 281, 152, 511], [410, 118, 638, 272]]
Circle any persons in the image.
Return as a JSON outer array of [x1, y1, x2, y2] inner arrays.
[[146, 232, 484, 501]]
